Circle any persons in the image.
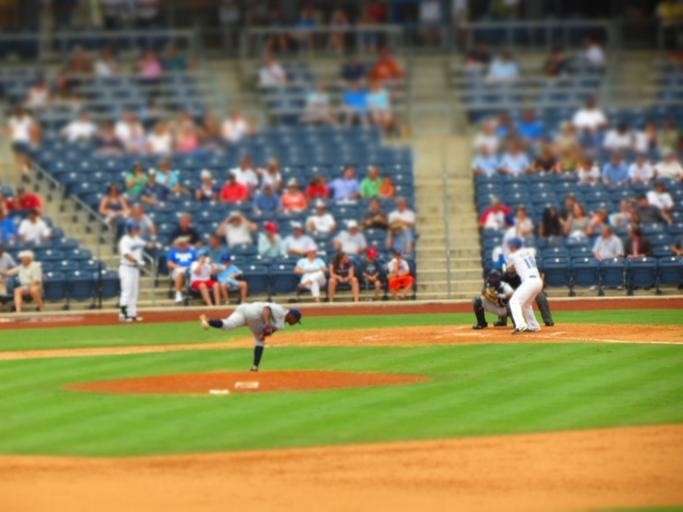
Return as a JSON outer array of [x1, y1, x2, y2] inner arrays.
[[200, 303, 302, 373]]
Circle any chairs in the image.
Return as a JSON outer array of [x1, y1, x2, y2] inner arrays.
[[0, 124, 682, 293]]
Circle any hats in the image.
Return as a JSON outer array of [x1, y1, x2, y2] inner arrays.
[[0, 180, 43, 259], [102, 155, 409, 263], [474, 107, 682, 279], [290, 308, 302, 325]]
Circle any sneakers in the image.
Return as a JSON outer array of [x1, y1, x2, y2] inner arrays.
[[118, 312, 144, 324], [251, 366, 258, 371], [199, 314, 209, 331], [473, 320, 554, 333]]
